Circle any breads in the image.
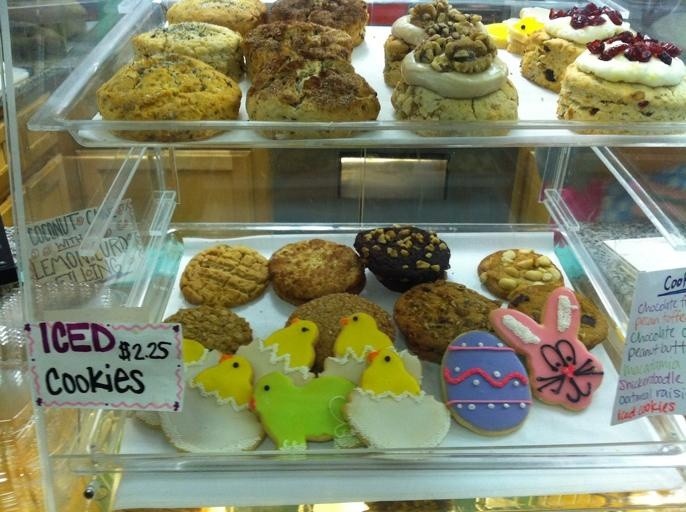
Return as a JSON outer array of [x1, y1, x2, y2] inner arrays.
[[96, 0, 686, 141]]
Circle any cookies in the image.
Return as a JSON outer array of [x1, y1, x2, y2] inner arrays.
[[125, 225, 609, 461]]
